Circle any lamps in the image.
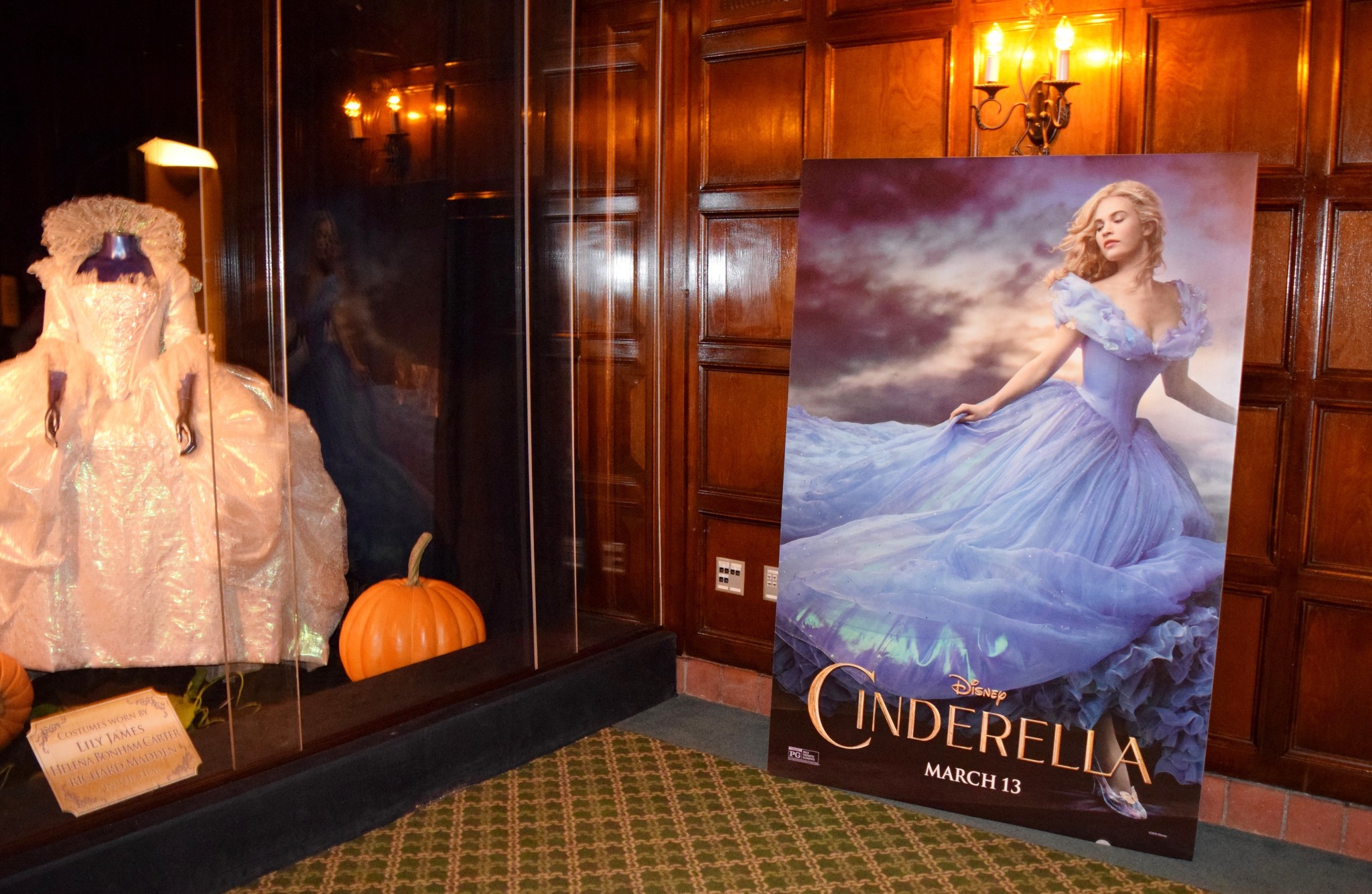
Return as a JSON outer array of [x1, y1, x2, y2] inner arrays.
[[970, 16, 1080, 155], [345, 92, 412, 177]]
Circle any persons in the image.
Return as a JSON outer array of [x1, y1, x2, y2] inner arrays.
[[286, 214, 437, 569], [775, 178, 1241, 820]]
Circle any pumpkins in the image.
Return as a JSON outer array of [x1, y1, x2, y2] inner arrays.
[[338, 533, 486, 682], [0, 652, 34, 752]]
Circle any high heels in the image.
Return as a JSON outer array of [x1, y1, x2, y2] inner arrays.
[[1090, 754, 1148, 819]]
